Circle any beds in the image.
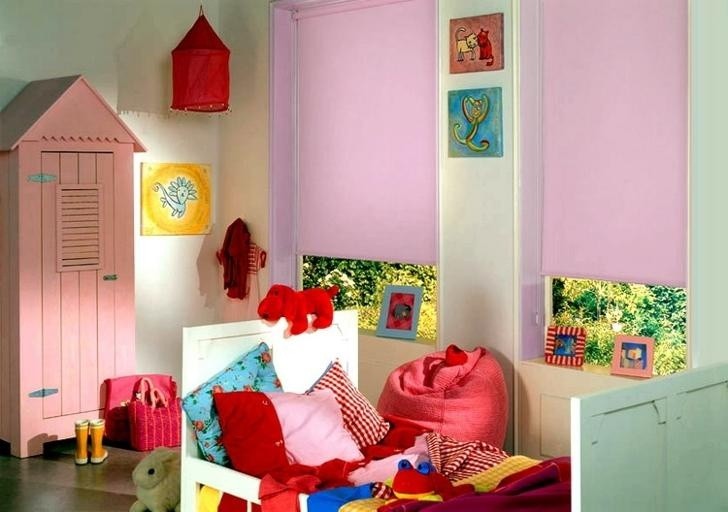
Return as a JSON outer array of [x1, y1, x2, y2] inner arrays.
[[173, 310, 727, 512]]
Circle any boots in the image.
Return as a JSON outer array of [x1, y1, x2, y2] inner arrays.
[[74, 420, 90, 463], [91, 419, 107, 463]]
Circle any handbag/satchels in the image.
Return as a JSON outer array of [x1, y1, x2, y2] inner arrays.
[[128, 378, 183, 452]]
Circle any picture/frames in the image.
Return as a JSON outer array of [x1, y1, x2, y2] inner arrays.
[[447, 87, 504, 158], [448, 12, 505, 74], [610, 333, 654, 379], [140, 162, 213, 237], [375, 284, 424, 341], [545, 324, 587, 368]]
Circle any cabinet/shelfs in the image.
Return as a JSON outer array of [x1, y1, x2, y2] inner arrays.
[[0, 73, 145, 460]]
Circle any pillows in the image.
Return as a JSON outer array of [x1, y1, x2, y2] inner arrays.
[[302, 357, 391, 451], [179, 342, 286, 466], [213, 389, 367, 476]]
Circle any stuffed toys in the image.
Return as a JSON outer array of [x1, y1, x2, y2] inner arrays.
[[130, 449, 182, 508], [257, 284, 338, 334], [392, 459, 474, 504]]
